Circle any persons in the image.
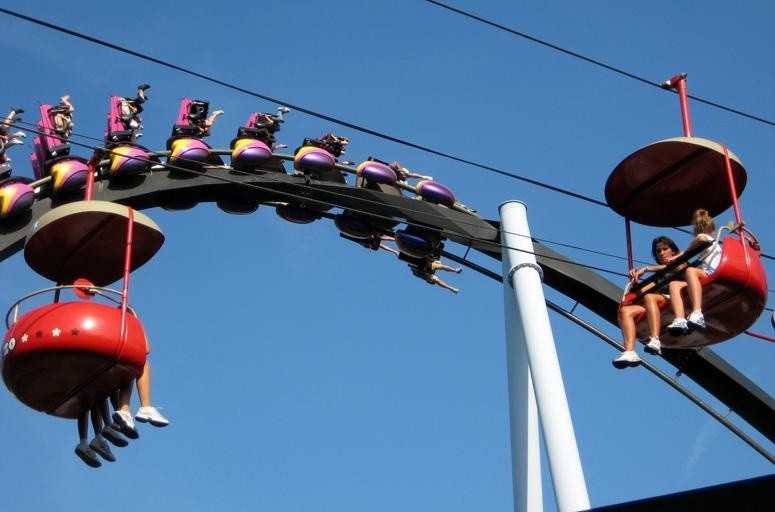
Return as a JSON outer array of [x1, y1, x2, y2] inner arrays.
[[392, 162, 477, 214], [0, 106, 27, 167], [267, 201, 288, 206], [427, 262, 463, 293], [254, 107, 291, 163], [319, 131, 355, 176], [667, 206, 724, 335], [56, 95, 75, 138], [71, 350, 170, 468], [197, 110, 224, 136], [123, 84, 150, 137], [610, 235, 688, 369], [380, 234, 398, 255]]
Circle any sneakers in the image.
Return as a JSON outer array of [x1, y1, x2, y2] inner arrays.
[[669, 318, 688, 332], [10, 108, 27, 146], [138, 83, 150, 91], [644, 338, 661, 355], [424, 175, 433, 181], [456, 267, 462, 274], [612, 351, 642, 369], [278, 107, 290, 114], [453, 289, 459, 293], [280, 144, 288, 149], [135, 406, 169, 428], [689, 313, 706, 328], [74, 406, 139, 468], [214, 110, 224, 115], [346, 160, 355, 165]]
